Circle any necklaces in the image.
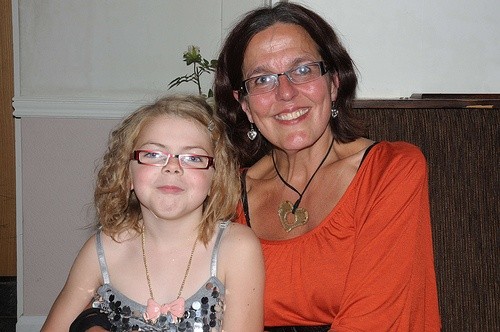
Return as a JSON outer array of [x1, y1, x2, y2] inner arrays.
[[140, 221, 201, 321], [271, 137, 335, 233]]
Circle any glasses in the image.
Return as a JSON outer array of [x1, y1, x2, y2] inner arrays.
[[129, 150, 213, 170], [239, 61, 330, 96]]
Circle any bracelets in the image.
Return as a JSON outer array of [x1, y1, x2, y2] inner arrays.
[[69, 308, 117, 332]]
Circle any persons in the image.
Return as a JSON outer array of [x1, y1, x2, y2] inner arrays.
[[40, 96, 265, 332], [69, 5, 442, 332]]
